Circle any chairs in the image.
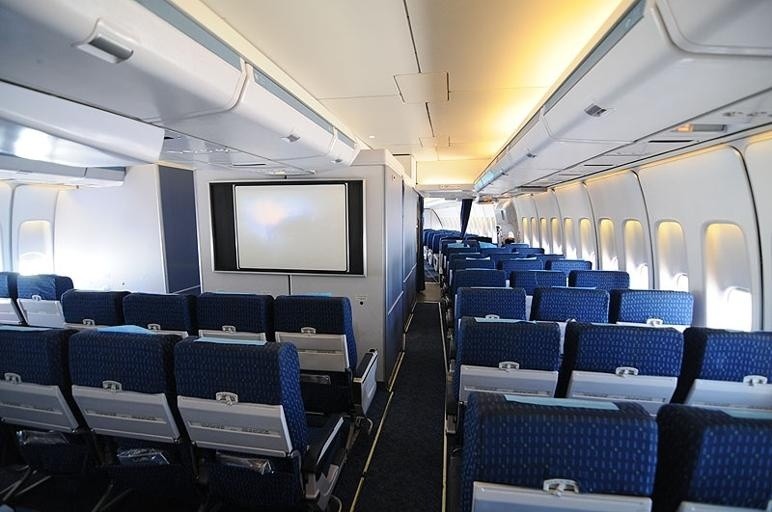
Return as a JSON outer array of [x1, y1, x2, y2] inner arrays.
[[173, 336, 356, 511], [15, 274, 74, 326], [67, 332, 185, 512], [271, 294, 378, 453], [0, 271, 23, 324], [59, 287, 127, 328], [0, 324, 93, 511], [121, 293, 195, 333], [195, 292, 273, 341]]
